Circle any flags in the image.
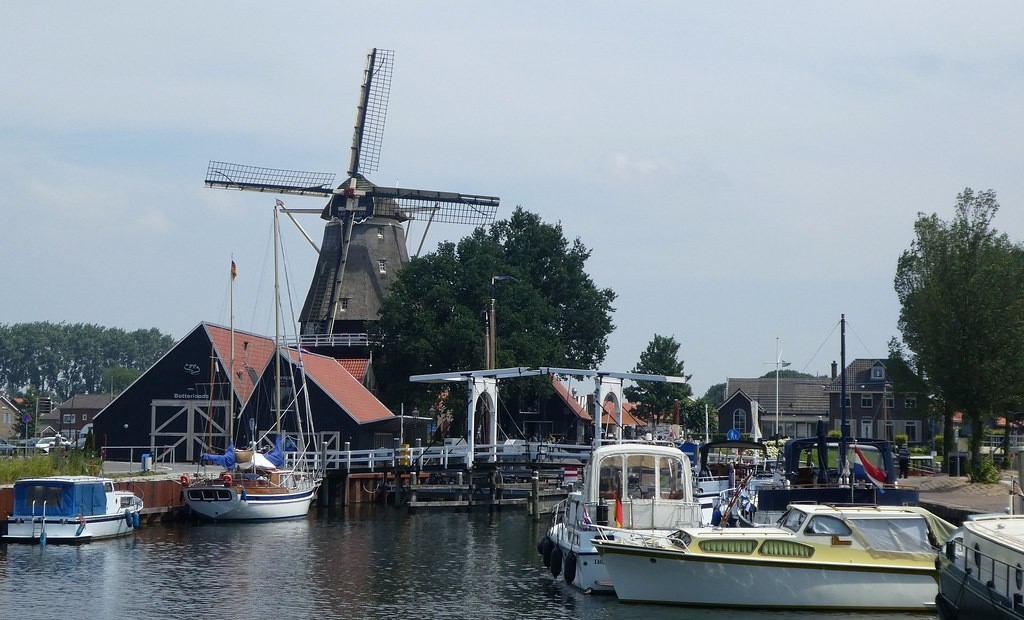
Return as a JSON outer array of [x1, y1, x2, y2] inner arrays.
[[614, 479, 624, 527], [854, 446, 887, 493], [581, 502, 593, 524]]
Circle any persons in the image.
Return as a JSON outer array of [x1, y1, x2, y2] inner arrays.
[[897, 445, 910, 478]]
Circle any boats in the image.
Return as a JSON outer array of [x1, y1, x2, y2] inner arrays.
[[536, 313, 1024, 620], [1, 475, 145, 544]]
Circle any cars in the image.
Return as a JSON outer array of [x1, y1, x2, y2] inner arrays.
[[0, 438, 19, 457], [17, 436, 87, 455]]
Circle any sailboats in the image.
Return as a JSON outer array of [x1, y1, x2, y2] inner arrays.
[[179, 198, 323, 522]]
